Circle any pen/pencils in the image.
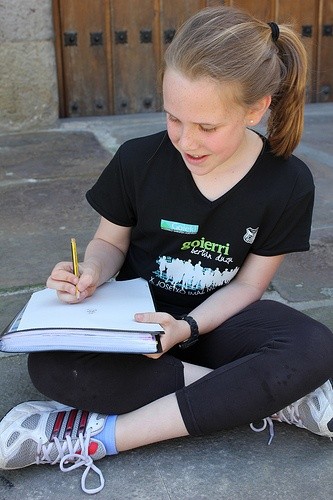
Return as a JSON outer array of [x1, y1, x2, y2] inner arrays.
[[70, 238, 81, 298]]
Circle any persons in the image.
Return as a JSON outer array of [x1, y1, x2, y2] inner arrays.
[[1, 8, 333, 471]]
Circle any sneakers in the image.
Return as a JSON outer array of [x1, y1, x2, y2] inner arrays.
[[249, 376, 333, 446], [0, 398, 109, 494]]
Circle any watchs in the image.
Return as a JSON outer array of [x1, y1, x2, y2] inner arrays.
[[176, 314, 200, 351]]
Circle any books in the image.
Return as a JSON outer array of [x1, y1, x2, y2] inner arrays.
[[0, 277, 165, 354]]
